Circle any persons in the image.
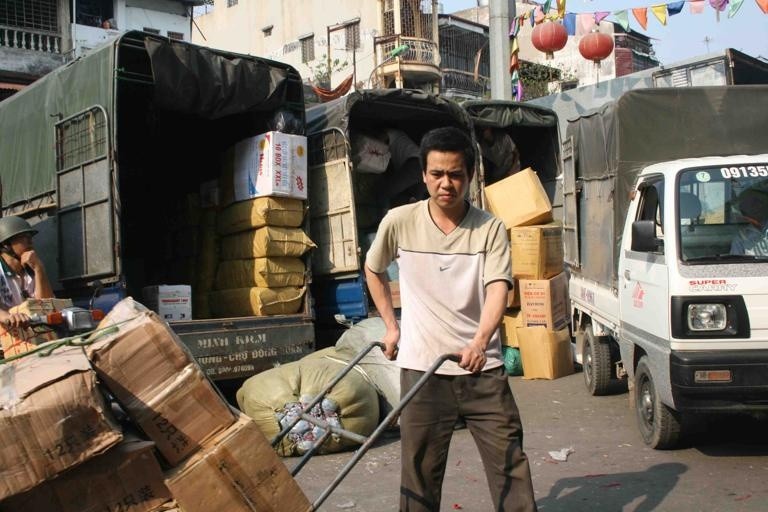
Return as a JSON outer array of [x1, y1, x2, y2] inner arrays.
[[363, 125, 538, 512], [101, 20, 113, 29], [729, 188, 768, 257], [0, 216, 57, 329]]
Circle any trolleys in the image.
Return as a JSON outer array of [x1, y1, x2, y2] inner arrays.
[[269, 341, 461, 511]]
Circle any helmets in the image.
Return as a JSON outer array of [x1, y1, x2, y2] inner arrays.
[[0, 216, 39, 243]]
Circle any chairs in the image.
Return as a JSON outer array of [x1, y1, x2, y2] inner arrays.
[[679, 192, 701, 225]]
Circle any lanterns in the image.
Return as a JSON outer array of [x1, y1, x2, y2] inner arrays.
[[530, 21, 569, 61], [578, 29, 615, 68]]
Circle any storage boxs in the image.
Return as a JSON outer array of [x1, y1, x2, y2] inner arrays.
[[483, 166, 575, 380], [232, 130, 308, 202], [388, 281, 401, 309]]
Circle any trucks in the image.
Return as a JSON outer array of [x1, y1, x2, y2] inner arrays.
[[562, 83, 768, 449]]
